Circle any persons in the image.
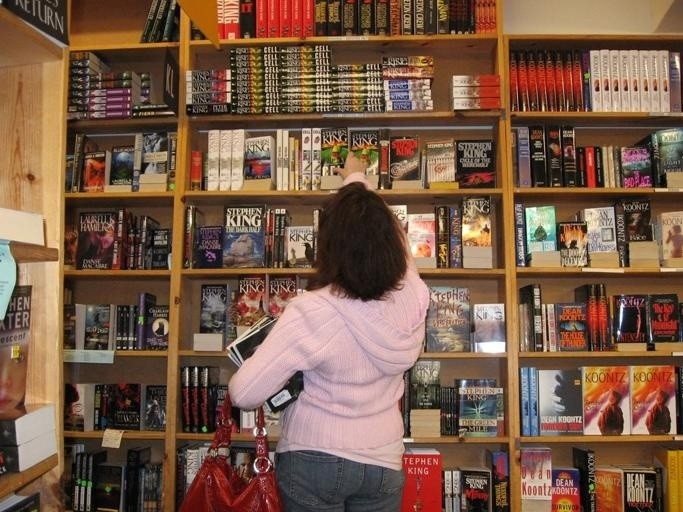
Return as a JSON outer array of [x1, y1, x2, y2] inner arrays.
[[0, 343, 27, 411], [228, 151, 430, 512]]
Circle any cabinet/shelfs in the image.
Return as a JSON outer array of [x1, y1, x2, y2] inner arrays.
[[69, 41, 168, 512], [503, 33, 683, 512], [1, 1, 68, 511], [171, 0, 516, 511]]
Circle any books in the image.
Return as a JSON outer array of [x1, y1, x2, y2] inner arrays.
[[0, 284, 35, 411], [0, 491, 41, 512], [63, 274, 304, 512], [0, 429, 58, 473], [0, 402, 55, 448], [64, 1, 683, 271], [398, 283, 683, 512]]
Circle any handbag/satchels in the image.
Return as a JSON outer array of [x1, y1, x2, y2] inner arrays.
[[179, 455, 285, 512]]
